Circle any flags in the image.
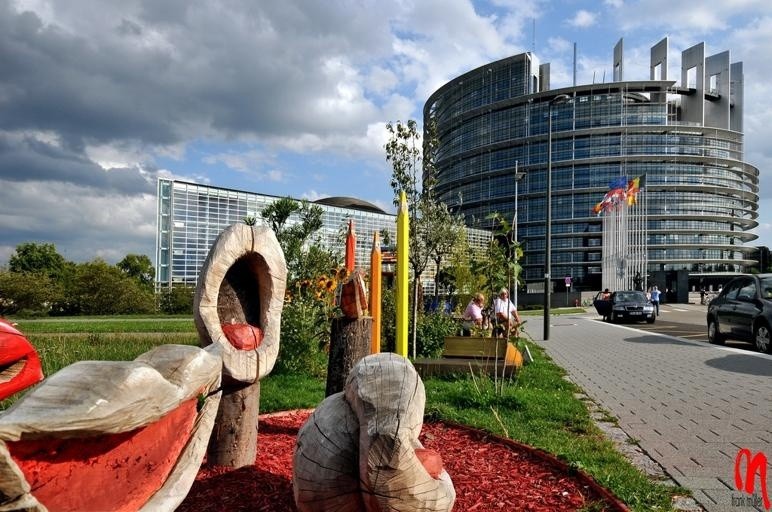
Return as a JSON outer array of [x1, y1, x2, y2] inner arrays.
[[594, 173, 645, 214]]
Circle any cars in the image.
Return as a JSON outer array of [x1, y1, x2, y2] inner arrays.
[[592, 287, 657, 325], [706, 273, 771, 358]]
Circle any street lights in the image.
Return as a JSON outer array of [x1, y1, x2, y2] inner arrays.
[[545, 91, 572, 338]]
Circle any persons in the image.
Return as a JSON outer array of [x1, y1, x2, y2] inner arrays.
[[602, 288, 610, 321], [650, 285, 662, 318], [459, 291, 492, 331], [699, 285, 708, 304], [489, 287, 524, 338]]
